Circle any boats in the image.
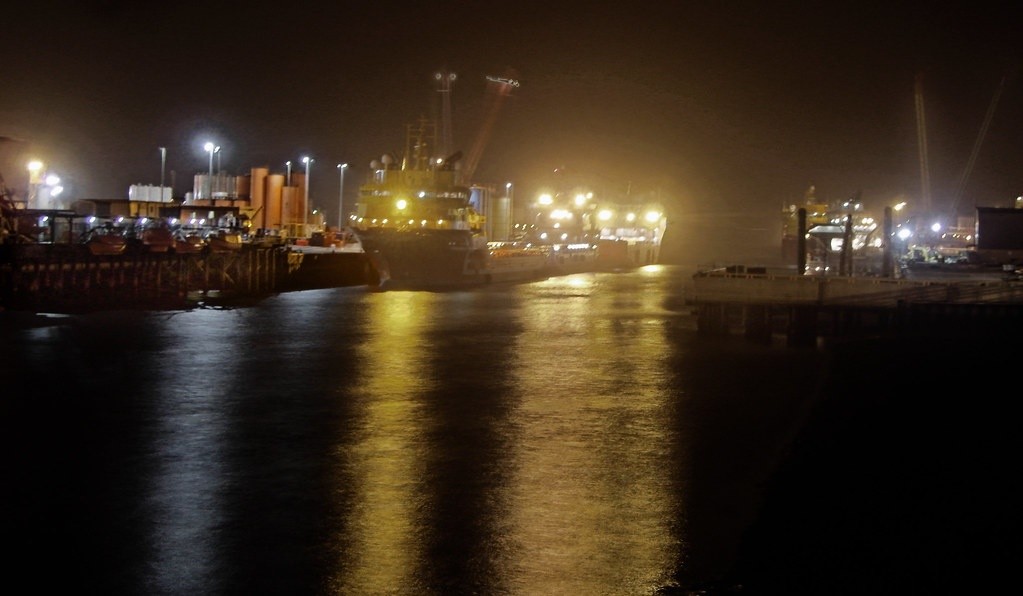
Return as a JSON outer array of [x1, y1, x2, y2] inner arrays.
[[348, 114, 668, 290]]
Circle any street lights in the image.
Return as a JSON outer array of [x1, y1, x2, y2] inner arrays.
[[286, 160, 292, 186], [213, 145, 221, 198], [204, 143, 213, 199], [302, 157, 311, 226], [338, 163, 348, 234]]
